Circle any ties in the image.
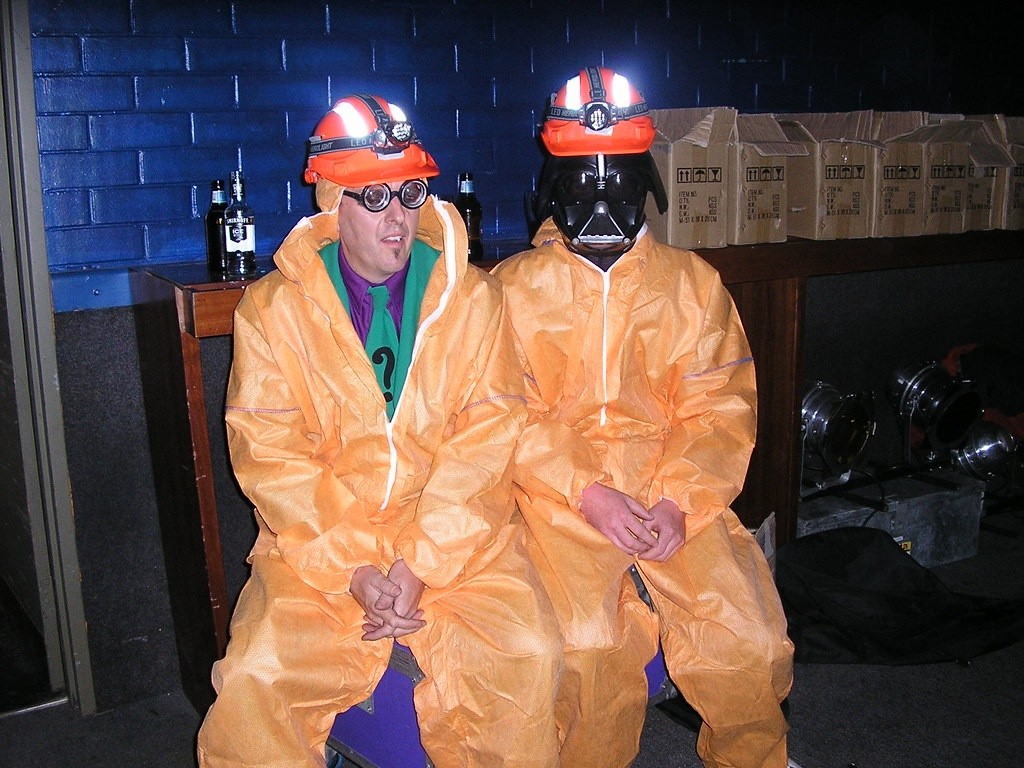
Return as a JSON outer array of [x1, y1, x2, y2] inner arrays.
[[365, 285, 400, 422]]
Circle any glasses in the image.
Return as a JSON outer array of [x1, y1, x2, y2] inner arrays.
[[343, 179, 428, 212]]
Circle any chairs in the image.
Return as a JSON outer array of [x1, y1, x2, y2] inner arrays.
[[324, 627, 683, 768]]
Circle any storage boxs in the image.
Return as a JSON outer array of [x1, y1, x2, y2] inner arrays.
[[797, 470, 989, 566], [646, 103, 1024, 251]]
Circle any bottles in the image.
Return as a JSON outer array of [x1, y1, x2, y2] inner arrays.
[[455, 172, 485, 261], [224, 170, 256, 280], [205, 180, 229, 281]]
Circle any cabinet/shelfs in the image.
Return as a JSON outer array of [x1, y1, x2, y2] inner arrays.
[[130, 234, 1024, 714]]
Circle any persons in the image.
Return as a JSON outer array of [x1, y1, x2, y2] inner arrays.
[[195, 95, 566, 768], [489, 68, 803, 768]]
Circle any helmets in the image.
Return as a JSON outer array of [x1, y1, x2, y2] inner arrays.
[[540, 66, 656, 156], [304, 93, 441, 188]]
